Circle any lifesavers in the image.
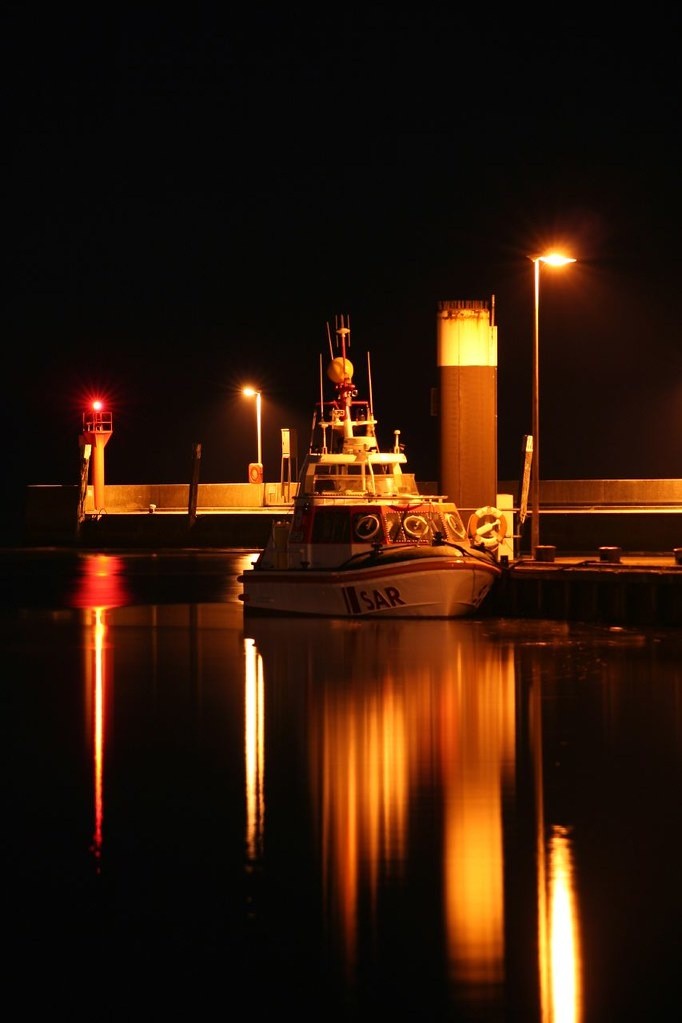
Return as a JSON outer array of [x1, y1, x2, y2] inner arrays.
[[470, 506, 507, 546]]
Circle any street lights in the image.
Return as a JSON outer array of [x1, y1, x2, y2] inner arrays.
[[245, 387, 262, 464], [527, 252, 578, 560]]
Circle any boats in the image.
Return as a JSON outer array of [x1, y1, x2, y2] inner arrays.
[[238, 313, 503, 619]]
